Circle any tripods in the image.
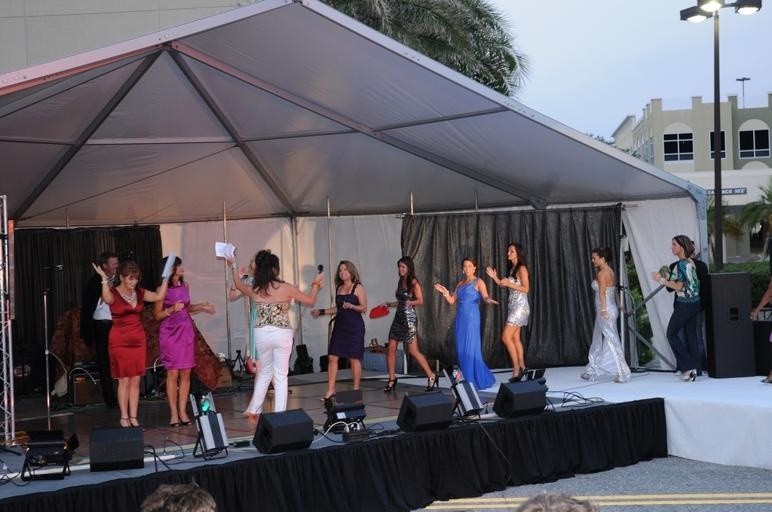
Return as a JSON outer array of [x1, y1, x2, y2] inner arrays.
[[232, 348, 250, 378]]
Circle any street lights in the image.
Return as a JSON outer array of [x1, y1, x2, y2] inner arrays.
[[678, 0, 763, 273]]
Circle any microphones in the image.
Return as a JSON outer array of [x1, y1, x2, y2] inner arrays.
[[316, 264, 323, 276]]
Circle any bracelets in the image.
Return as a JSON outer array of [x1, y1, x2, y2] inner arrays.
[[443, 291, 449, 296], [385, 302, 391, 307], [230, 288, 236, 291], [165, 308, 171, 317], [600, 308, 608, 312], [101, 280, 108, 284], [349, 304, 353, 309], [319, 309, 325, 315], [312, 281, 321, 288], [162, 279, 169, 283], [658, 278, 665, 285], [231, 265, 240, 271]]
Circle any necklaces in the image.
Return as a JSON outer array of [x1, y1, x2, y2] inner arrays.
[[118, 288, 137, 304]]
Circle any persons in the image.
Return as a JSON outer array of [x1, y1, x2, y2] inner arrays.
[[311, 261, 367, 402], [750, 277, 772, 383], [652, 240, 711, 379], [153, 256, 217, 426], [227, 259, 257, 359], [380, 256, 439, 393], [651, 236, 702, 383], [90, 262, 174, 432], [581, 248, 630, 383], [218, 250, 325, 417], [433, 256, 500, 390], [79, 252, 119, 410], [486, 242, 531, 382]]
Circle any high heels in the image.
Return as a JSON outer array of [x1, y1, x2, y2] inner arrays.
[[509, 367, 523, 383], [129, 416, 146, 432], [119, 417, 132, 429], [384, 377, 398, 392], [682, 367, 698, 382], [169, 414, 180, 427], [424, 374, 439, 391], [178, 413, 191, 425], [762, 376, 772, 383]]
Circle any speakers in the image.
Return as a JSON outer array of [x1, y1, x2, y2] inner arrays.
[[704, 271, 757, 378], [395, 391, 453, 433], [493, 377, 549, 419], [251, 408, 316, 453], [85, 425, 146, 472]]
